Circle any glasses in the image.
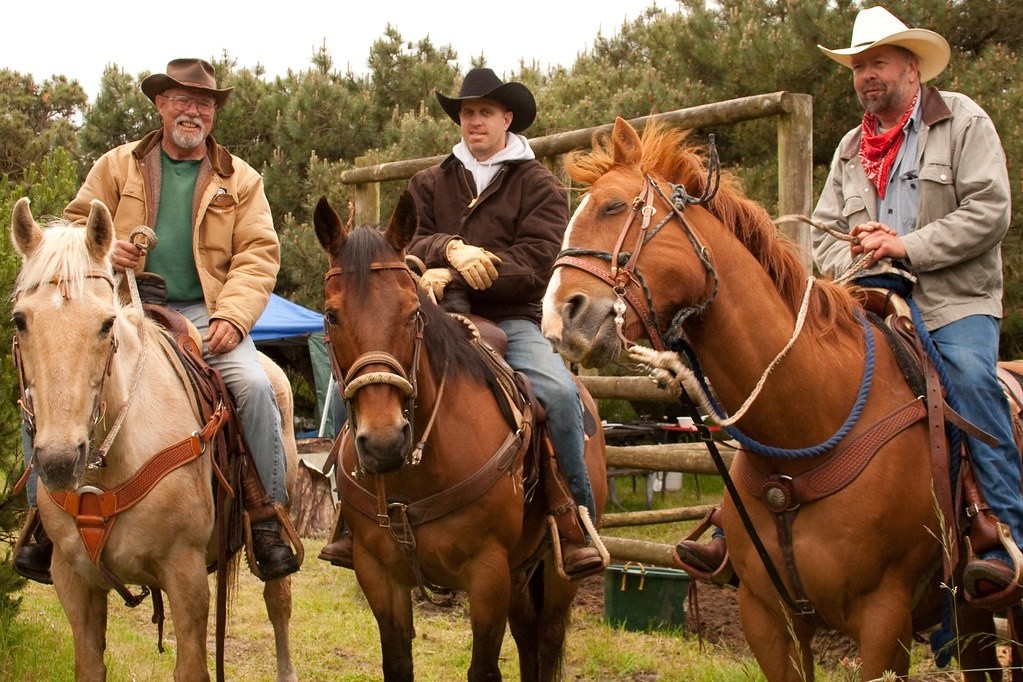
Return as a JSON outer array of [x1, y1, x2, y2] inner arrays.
[[158, 94, 218, 110]]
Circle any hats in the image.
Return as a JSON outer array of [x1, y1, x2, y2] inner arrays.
[[434, 68, 536, 133], [815, 4, 950, 83], [141, 57, 235, 107]]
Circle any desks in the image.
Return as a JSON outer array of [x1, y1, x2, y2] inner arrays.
[[602, 424, 727, 500]]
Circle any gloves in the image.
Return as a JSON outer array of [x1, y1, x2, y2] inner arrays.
[[416, 268, 451, 301], [445, 239, 503, 290]]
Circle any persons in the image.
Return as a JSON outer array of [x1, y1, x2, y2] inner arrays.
[[12, 58, 299, 580], [675, 7, 1023, 605], [313, 70, 604, 573]]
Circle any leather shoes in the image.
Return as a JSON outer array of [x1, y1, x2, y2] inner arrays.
[[14, 539, 52, 573], [250, 529, 299, 578], [553, 536, 601, 574], [675, 536, 733, 583], [963, 558, 1023, 616], [318, 533, 354, 567]]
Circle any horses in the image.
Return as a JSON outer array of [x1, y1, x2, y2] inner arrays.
[[9, 194, 301, 682], [312, 190, 607, 682], [540, 117, 1022, 682]]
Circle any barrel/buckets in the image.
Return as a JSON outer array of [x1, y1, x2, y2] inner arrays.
[[603, 561, 690, 634]]
